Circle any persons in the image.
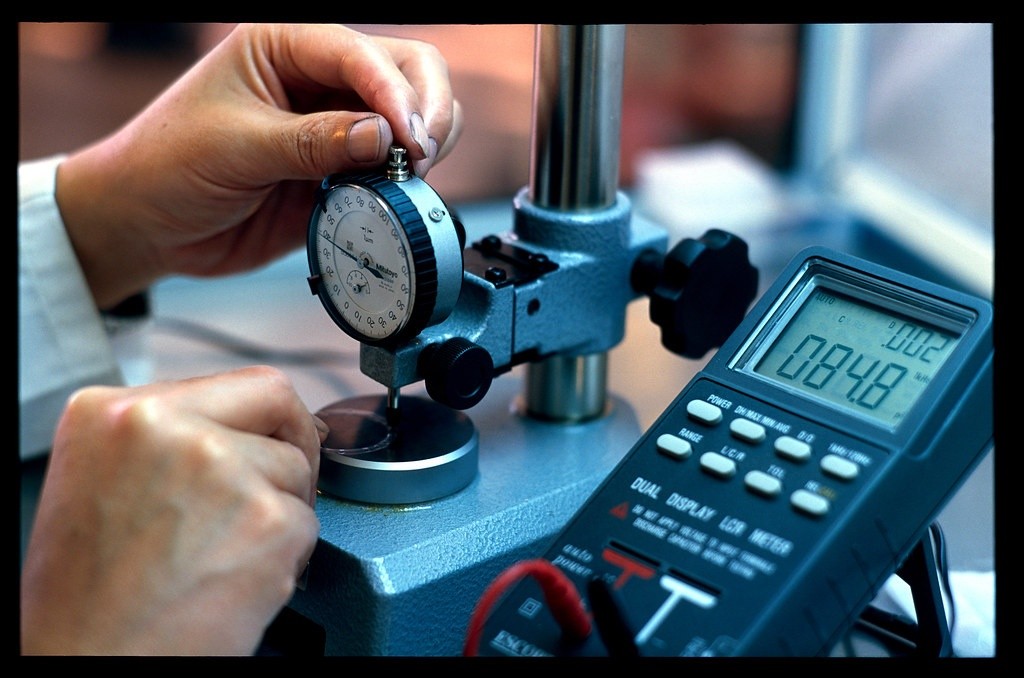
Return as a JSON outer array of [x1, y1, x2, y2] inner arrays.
[[18, 23, 464, 656]]
